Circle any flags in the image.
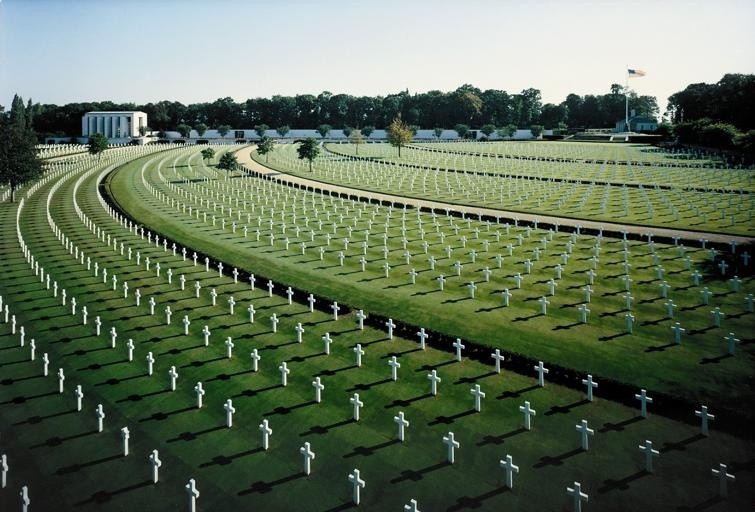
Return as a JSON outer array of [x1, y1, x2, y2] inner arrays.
[[628, 69, 647, 78]]
